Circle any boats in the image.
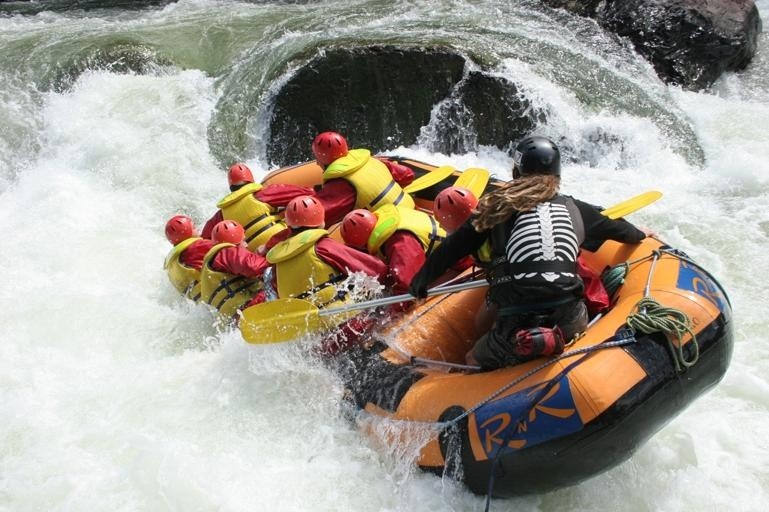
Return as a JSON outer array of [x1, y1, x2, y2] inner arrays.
[[253, 156, 737, 502]]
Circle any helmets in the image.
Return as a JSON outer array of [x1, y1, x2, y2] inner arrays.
[[211, 219, 243, 246], [166, 216, 192, 245], [513, 138, 560, 175], [434, 187, 479, 229], [227, 163, 254, 191], [286, 196, 325, 227], [341, 209, 378, 247], [312, 131, 347, 164]]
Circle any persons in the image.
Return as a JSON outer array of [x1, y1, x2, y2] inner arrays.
[[202, 162, 318, 256], [432, 185, 613, 335], [338, 201, 473, 313], [310, 132, 419, 229], [162, 213, 218, 307], [406, 132, 658, 373], [263, 195, 387, 364], [196, 220, 279, 321]]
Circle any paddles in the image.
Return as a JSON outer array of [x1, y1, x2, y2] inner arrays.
[[401, 165, 455, 194], [239, 278, 488, 346], [600, 189, 663, 223], [453, 168, 490, 200]]
